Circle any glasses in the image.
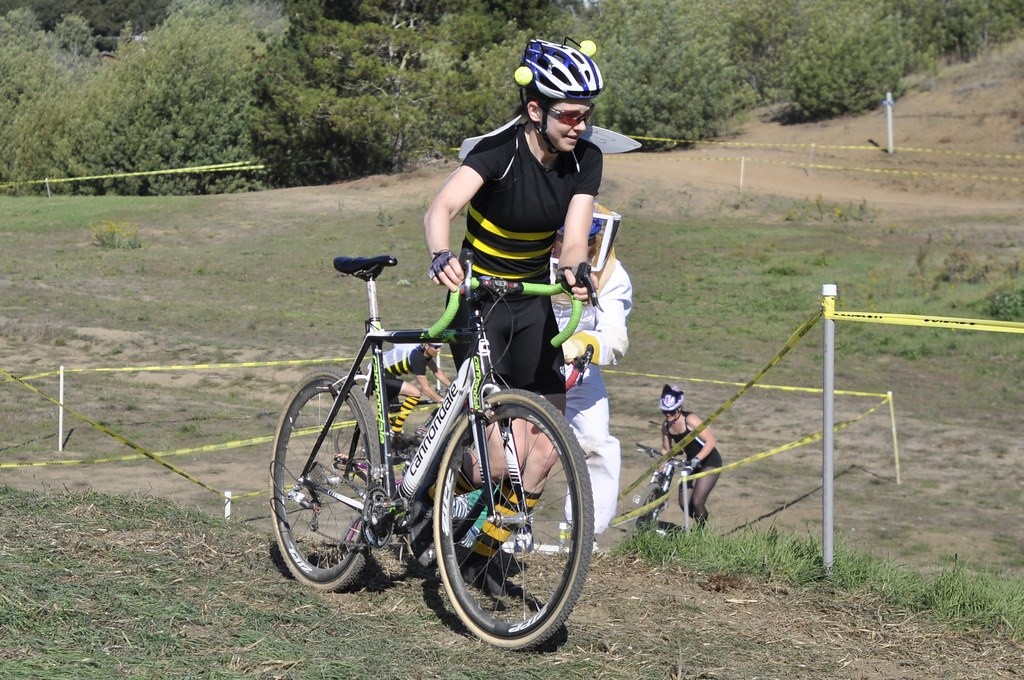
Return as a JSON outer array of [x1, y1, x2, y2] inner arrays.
[[429, 345, 442, 348], [555, 235, 596, 246], [660, 409, 676, 416], [531, 95, 595, 126]]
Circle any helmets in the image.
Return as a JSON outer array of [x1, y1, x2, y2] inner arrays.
[[658, 384, 684, 410], [558, 209, 602, 236], [520, 38, 604, 99]]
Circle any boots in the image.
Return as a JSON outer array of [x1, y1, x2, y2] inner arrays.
[[407, 448, 479, 565], [461, 480, 544, 598]]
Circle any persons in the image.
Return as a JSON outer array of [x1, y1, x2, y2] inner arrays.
[[656, 386, 722, 538], [366, 334, 454, 453], [421, 47, 604, 601], [543, 210, 632, 561]]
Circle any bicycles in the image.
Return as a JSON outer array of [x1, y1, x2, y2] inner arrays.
[[632, 439, 703, 539], [332, 384, 452, 465], [266, 245, 604, 654]]
